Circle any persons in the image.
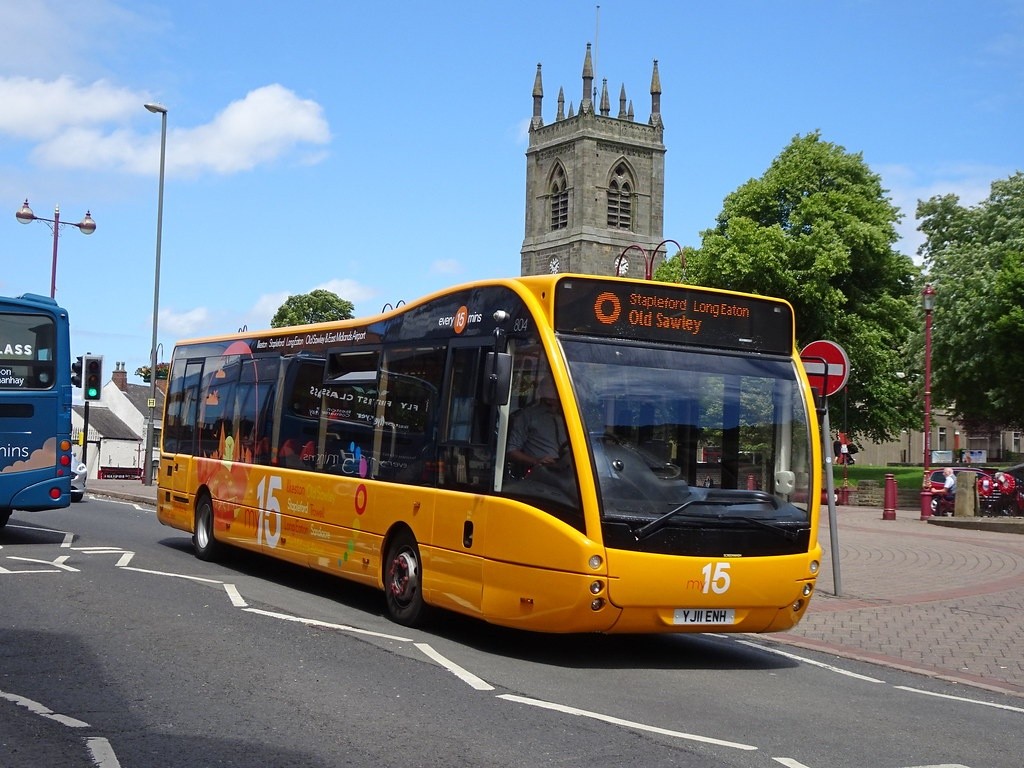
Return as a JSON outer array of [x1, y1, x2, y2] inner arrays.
[[931, 467, 957, 516], [507, 374, 569, 469]]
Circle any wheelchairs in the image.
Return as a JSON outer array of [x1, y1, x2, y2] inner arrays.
[[930, 489, 955, 516]]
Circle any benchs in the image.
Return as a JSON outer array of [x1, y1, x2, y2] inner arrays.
[[939, 476, 1017, 516]]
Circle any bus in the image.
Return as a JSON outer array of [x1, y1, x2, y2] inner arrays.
[[0, 294, 71, 528], [168, 357, 437, 489], [157, 273, 828, 634]]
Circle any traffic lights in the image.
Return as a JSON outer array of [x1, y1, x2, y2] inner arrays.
[[82, 355, 103, 401], [71, 357, 82, 388]]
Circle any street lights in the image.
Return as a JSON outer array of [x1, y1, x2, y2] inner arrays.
[[144, 104, 168, 488], [16, 198, 96, 298], [921, 284, 937, 517]]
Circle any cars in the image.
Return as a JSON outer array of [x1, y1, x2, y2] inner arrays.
[[71, 454, 87, 503], [980, 464, 1024, 516], [929, 468, 993, 496]]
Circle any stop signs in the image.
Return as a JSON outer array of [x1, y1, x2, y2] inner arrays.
[[800, 340, 851, 398]]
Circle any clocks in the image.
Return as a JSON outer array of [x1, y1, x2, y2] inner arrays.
[[614, 256, 630, 275], [550, 257, 559, 274]]
